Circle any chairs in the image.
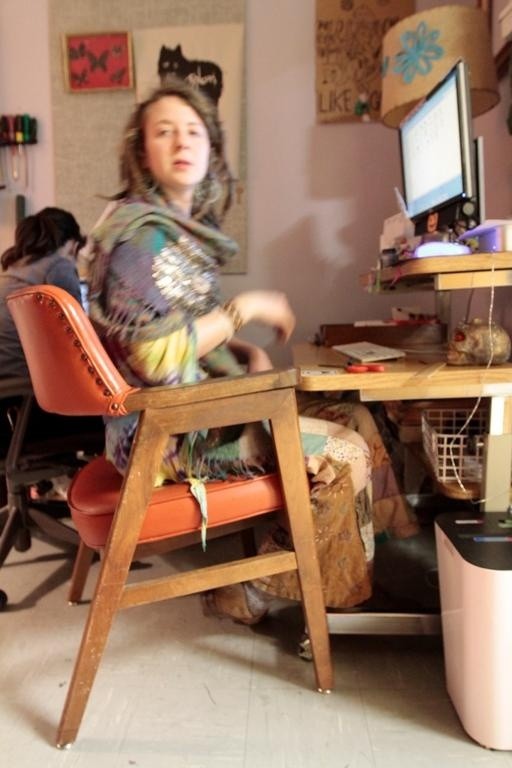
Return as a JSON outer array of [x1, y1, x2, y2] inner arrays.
[[3, 283, 108, 609], [5, 286, 333, 750]]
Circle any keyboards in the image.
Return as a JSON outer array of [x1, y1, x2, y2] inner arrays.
[[332, 340, 407, 366]]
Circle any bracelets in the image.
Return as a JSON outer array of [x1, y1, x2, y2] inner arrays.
[[218, 301, 245, 334]]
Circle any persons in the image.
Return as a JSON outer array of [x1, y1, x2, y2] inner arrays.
[[77, 84, 419, 629], [1, 205, 104, 497]]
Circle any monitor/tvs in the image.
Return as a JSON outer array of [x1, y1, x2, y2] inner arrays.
[[396, 58, 475, 222]]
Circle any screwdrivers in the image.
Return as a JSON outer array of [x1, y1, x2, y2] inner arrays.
[[1, 113, 31, 187]]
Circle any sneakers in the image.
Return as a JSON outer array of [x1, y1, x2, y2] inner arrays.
[[296, 628, 340, 662], [202, 581, 268, 625]]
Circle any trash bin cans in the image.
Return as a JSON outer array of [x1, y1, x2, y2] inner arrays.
[[434, 507, 511, 753]]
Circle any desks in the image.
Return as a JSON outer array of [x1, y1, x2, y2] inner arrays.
[[292, 336, 512, 660]]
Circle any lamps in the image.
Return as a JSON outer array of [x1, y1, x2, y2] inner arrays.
[[383, 5, 501, 129]]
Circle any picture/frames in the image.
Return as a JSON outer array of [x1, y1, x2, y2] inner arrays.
[[59, 31, 132, 94]]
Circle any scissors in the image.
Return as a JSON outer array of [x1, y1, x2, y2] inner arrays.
[[319, 363, 386, 374]]
